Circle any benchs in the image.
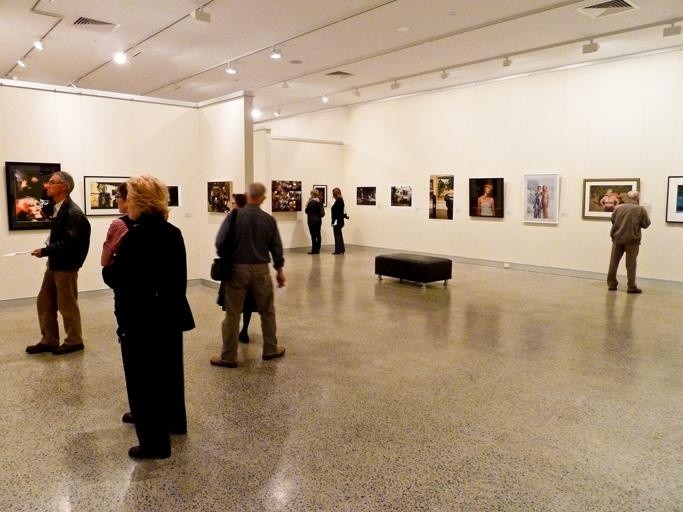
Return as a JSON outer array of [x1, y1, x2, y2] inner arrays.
[[375, 253, 452, 290]]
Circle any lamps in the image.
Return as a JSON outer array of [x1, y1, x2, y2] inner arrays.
[[18, 58, 28, 69], [662, 25, 682, 38], [226, 60, 237, 75], [111, 50, 134, 68], [391, 82, 402, 90], [272, 96, 331, 117], [441, 71, 449, 80], [282, 81, 289, 90], [190, 7, 212, 25], [582, 41, 599, 54], [35, 38, 46, 52], [272, 46, 283, 60], [503, 59, 512, 68], [349, 88, 361, 97]]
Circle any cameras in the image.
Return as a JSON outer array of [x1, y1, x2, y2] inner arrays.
[[344, 213, 349, 219]]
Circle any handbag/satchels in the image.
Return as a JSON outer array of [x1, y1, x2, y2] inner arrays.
[[212, 258, 236, 283]]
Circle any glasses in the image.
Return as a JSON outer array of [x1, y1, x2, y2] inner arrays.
[[46, 180, 64, 185], [115, 193, 122, 200]]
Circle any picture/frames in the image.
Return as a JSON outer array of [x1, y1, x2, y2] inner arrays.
[[522, 174, 559, 225], [469, 178, 504, 218], [84, 176, 130, 217], [666, 176, 683, 224], [582, 178, 640, 220], [5, 161, 61, 231], [313, 184, 327, 207]]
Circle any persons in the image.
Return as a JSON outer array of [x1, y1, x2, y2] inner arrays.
[[101, 182, 141, 423], [331, 187, 347, 255], [478, 183, 495, 217], [26, 172, 90, 356], [607, 191, 650, 294], [231, 194, 260, 342], [20, 198, 43, 220], [306, 189, 325, 253], [128, 176, 195, 459], [212, 184, 285, 367], [600, 189, 619, 212], [616, 192, 625, 204]]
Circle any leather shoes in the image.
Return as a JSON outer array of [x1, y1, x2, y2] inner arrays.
[[609, 286, 617, 290], [26, 343, 58, 354], [262, 347, 286, 359], [210, 355, 239, 368], [52, 343, 85, 355], [307, 249, 345, 255], [128, 445, 171, 459], [627, 287, 641, 293], [122, 413, 135, 423], [238, 332, 249, 343]]
